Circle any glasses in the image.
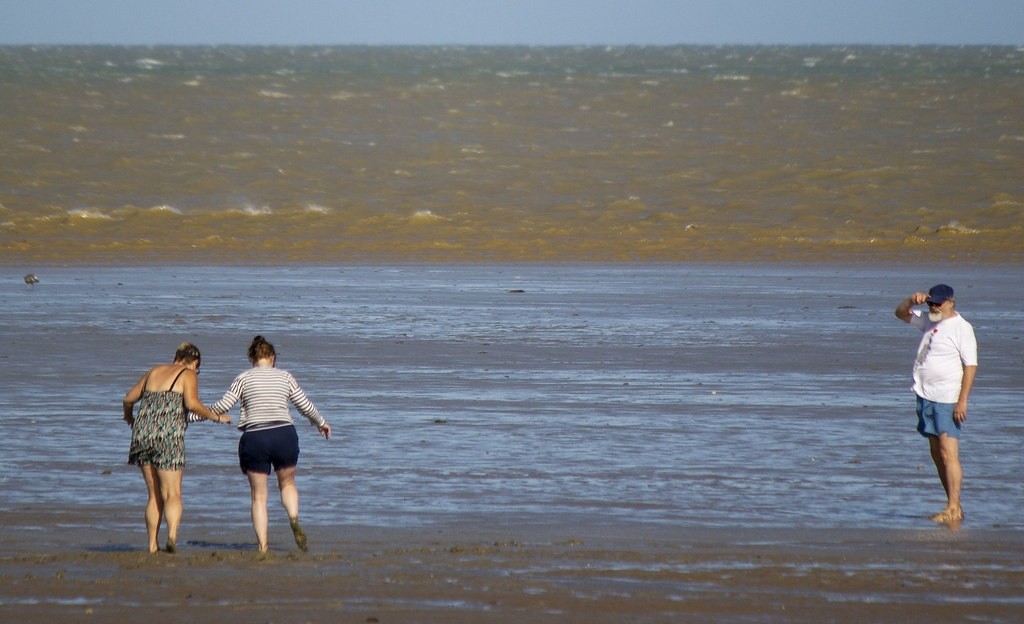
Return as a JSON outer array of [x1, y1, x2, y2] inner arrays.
[[197, 367, 200, 375], [927, 301, 943, 307]]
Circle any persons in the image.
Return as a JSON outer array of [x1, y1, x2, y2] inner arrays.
[[186, 335, 331, 553], [896, 284, 978, 521], [123, 342, 233, 552]]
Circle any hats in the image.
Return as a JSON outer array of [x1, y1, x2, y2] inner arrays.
[[928, 284, 954, 303]]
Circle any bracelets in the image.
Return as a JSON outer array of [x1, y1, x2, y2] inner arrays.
[[217, 414, 220, 422]]
